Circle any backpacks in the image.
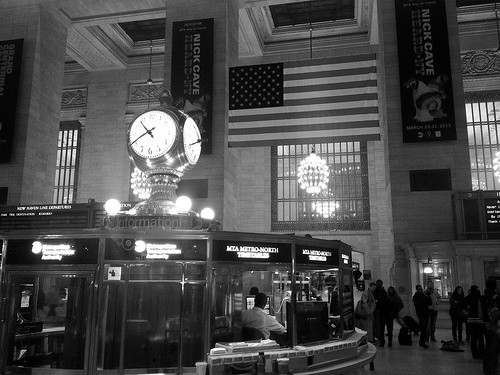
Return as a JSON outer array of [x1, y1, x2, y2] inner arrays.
[[440, 340, 465, 352], [399, 327, 413, 345]]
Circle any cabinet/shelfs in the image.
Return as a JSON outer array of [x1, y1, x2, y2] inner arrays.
[[208, 333, 377, 375]]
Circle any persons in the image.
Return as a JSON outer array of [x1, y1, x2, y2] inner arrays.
[[449, 286, 466, 344], [313, 271, 340, 316], [354, 290, 376, 370], [423, 281, 441, 342], [242, 294, 285, 340], [413, 285, 432, 349], [366, 280, 405, 347], [465, 277, 500, 375]]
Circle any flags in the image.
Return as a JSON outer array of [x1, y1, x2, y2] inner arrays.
[[228, 53, 381, 148]]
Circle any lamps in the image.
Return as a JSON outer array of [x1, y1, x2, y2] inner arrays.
[[201, 208, 215, 219], [104, 199, 121, 215], [176, 196, 192, 214]]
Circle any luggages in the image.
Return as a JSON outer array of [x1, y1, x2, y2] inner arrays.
[[397, 315, 420, 336]]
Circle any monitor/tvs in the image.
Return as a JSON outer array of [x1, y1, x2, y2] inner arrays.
[[246, 295, 270, 315], [286, 301, 330, 346]]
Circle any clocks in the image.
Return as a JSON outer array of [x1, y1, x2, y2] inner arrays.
[[126, 109, 180, 165], [177, 111, 203, 168]]
